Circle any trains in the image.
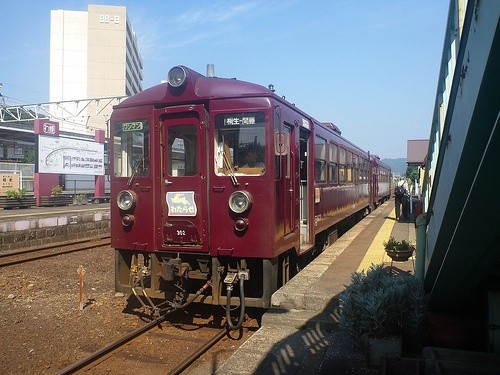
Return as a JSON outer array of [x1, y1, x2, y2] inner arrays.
[[108, 63, 394, 331]]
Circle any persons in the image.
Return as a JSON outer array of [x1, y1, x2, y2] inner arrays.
[[218, 138, 321, 183], [394, 177, 409, 221]]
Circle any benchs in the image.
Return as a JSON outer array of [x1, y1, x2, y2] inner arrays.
[[90, 196, 110, 204]]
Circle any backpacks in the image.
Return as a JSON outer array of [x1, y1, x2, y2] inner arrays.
[[394, 181, 406, 199]]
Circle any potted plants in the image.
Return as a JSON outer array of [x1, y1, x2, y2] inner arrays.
[[338, 263, 431, 367], [383, 236, 415, 261]]
